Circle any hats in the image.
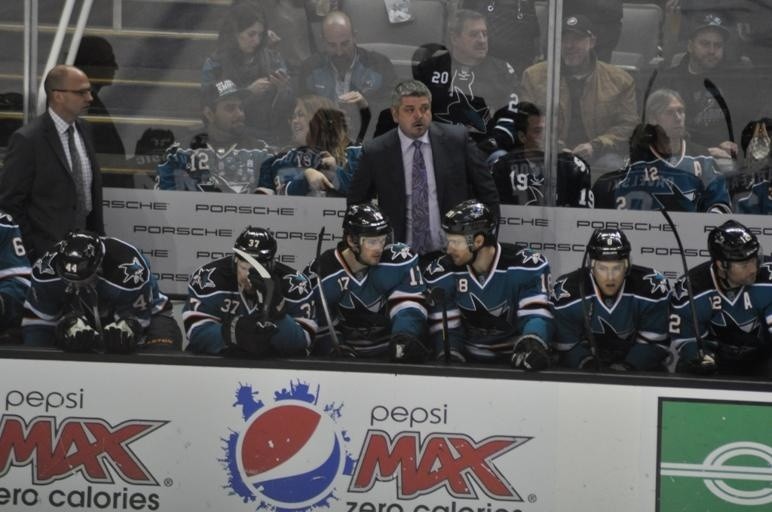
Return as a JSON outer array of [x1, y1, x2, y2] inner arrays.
[[687, 12, 730, 37], [199, 78, 253, 107], [557, 13, 596, 37]]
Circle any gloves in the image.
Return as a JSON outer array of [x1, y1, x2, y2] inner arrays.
[[327, 343, 359, 359], [221, 310, 305, 358], [609, 361, 638, 371], [108, 318, 139, 353], [385, 335, 428, 364], [691, 352, 717, 378], [566, 339, 603, 370], [512, 334, 553, 372], [59, 316, 102, 355]]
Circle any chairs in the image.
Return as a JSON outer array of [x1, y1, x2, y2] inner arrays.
[[265, 0, 772, 151]]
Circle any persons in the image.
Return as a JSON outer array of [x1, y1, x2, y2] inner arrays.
[[182, 226, 318, 356], [21, 231, 183, 355], [343, 82, 500, 255], [302, 205, 430, 365], [551, 228, 671, 373], [0, 209, 31, 347], [0, 65, 104, 262], [668, 221, 771, 376], [421, 199, 554, 369]]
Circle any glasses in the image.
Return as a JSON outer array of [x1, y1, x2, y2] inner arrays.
[[51, 87, 93, 96]]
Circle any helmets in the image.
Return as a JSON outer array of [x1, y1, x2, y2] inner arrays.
[[707, 219, 761, 282], [52, 228, 109, 296], [0, 90, 36, 149], [341, 202, 396, 255], [232, 224, 278, 293], [134, 128, 178, 168], [441, 199, 499, 264], [739, 116, 772, 168], [586, 225, 633, 284], [628, 122, 672, 163], [306, 107, 351, 150]]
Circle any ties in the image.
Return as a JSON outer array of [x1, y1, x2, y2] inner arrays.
[[410, 141, 433, 255], [67, 125, 89, 218]]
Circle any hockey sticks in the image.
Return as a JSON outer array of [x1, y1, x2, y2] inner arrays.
[[352, 89, 371, 144], [233, 247, 274, 325], [429, 286, 450, 364], [641, 69, 658, 122], [647, 192, 699, 349], [316, 226, 338, 346], [704, 79, 737, 168]]
